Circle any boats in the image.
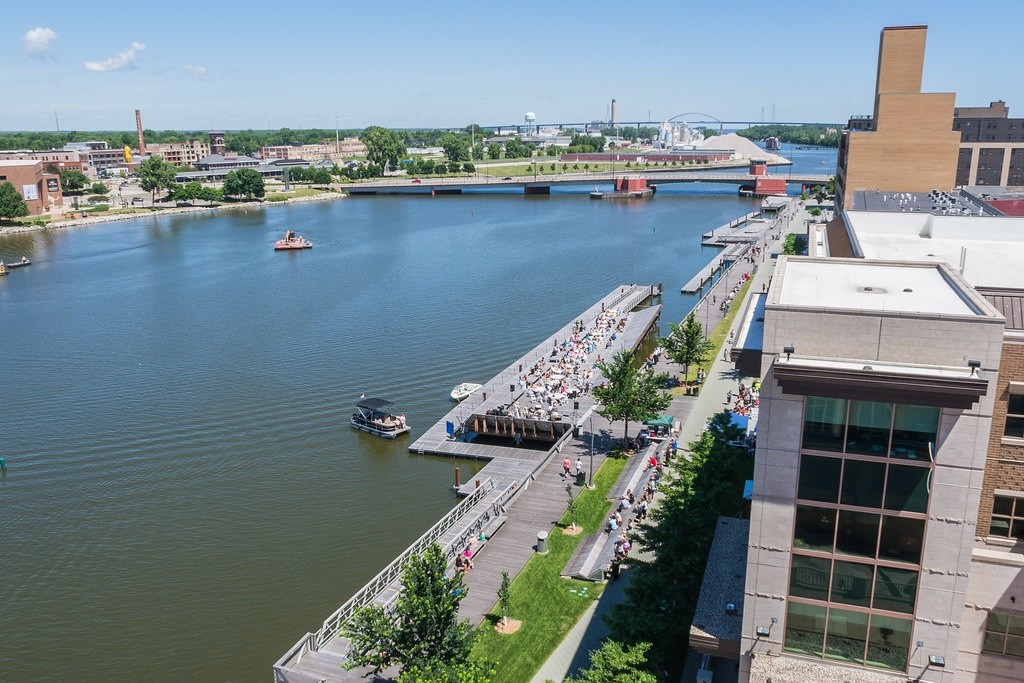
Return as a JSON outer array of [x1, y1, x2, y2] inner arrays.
[[451, 381, 484, 405], [273, 228, 315, 249], [348, 397, 412, 439]]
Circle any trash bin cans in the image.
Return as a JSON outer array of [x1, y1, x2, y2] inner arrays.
[[693, 387, 699, 397], [577, 471, 586, 486], [687, 388, 691, 395], [577, 427, 583, 435], [537, 530, 548, 553], [755, 265, 758, 271], [791, 217, 793, 221]]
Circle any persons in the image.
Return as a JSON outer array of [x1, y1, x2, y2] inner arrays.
[[727, 380, 760, 416], [456, 548, 474, 573], [646, 358, 652, 368], [724, 349, 728, 362], [730, 330, 734, 342], [608, 435, 678, 572], [697, 368, 705, 385], [479, 530, 485, 541], [563, 457, 582, 477], [712, 273, 750, 309], [513, 308, 632, 417]]
